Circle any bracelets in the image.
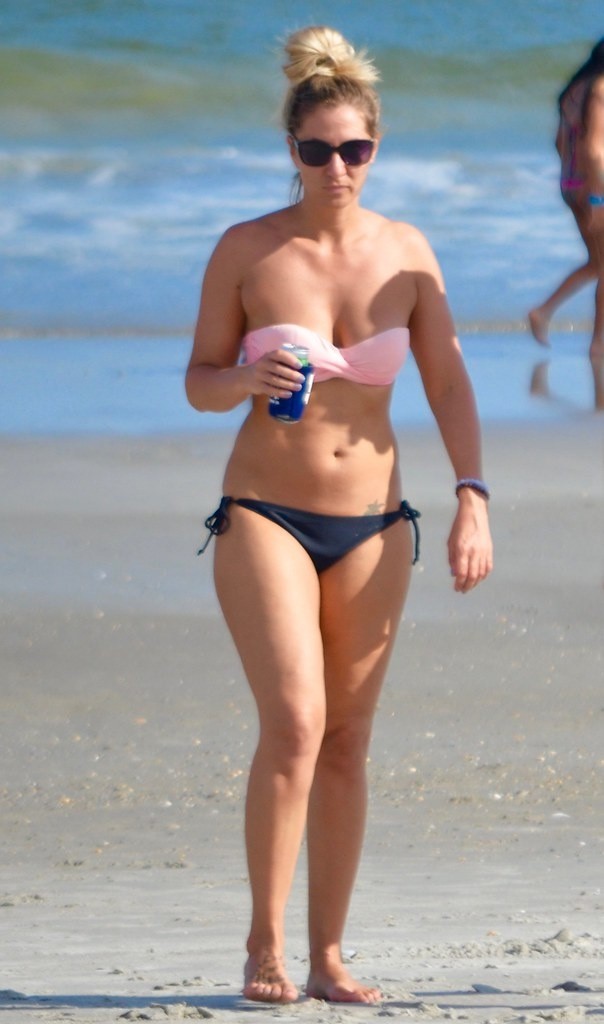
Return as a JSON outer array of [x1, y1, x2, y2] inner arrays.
[[452, 477, 491, 498]]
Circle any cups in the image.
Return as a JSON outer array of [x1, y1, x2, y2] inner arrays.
[[275, 343, 310, 425]]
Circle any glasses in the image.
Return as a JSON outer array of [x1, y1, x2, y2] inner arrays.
[[290, 133, 374, 167]]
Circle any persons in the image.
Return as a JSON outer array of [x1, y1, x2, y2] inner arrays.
[[185, 28, 494, 1007], [529, 38, 604, 356]]
